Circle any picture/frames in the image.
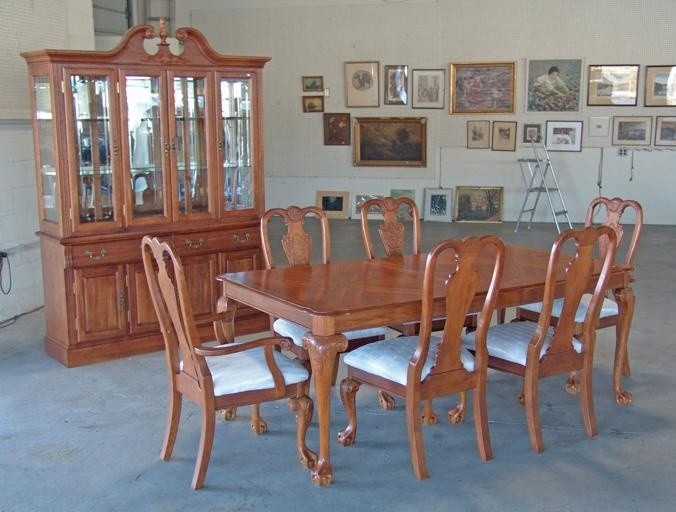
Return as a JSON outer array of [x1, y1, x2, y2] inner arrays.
[[302, 74, 325, 94], [611, 116, 652, 145], [645, 64, 676, 108], [412, 68, 446, 110], [353, 114, 428, 170], [587, 64, 640, 108], [467, 120, 490, 149], [423, 188, 453, 223], [301, 96, 326, 115], [343, 60, 381, 111], [526, 57, 584, 116], [352, 193, 390, 221], [590, 117, 610, 138], [455, 186, 503, 224], [491, 120, 517, 151], [323, 112, 352, 147], [384, 63, 408, 106], [655, 115, 676, 146], [315, 190, 349, 219], [523, 124, 541, 144], [546, 121, 583, 153], [449, 61, 516, 115]]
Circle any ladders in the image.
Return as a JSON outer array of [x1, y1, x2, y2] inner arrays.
[[513, 132, 574, 234]]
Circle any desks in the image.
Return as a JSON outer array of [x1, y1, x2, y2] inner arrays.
[[205, 236, 638, 484]]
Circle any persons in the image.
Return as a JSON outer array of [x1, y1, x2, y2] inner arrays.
[[534, 66, 570, 96]]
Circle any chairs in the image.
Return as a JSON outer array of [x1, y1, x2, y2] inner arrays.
[[256, 201, 398, 429], [357, 193, 480, 344], [437, 225, 622, 456], [327, 232, 508, 483], [508, 193, 644, 408], [136, 232, 321, 491]]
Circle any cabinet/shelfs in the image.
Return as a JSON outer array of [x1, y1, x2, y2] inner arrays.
[[170, 231, 268, 336], [39, 229, 179, 349], [23, 49, 170, 227], [170, 66, 263, 215]]
